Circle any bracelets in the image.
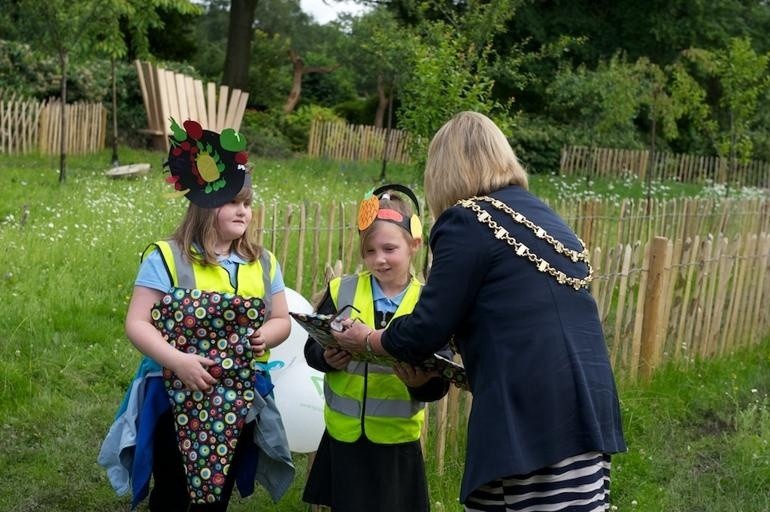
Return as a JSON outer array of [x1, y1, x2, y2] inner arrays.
[[363, 329, 384, 360]]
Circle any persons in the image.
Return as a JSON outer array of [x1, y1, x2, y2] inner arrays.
[[95, 163, 298, 511], [300, 184, 453, 511], [328, 109, 630, 512]]
